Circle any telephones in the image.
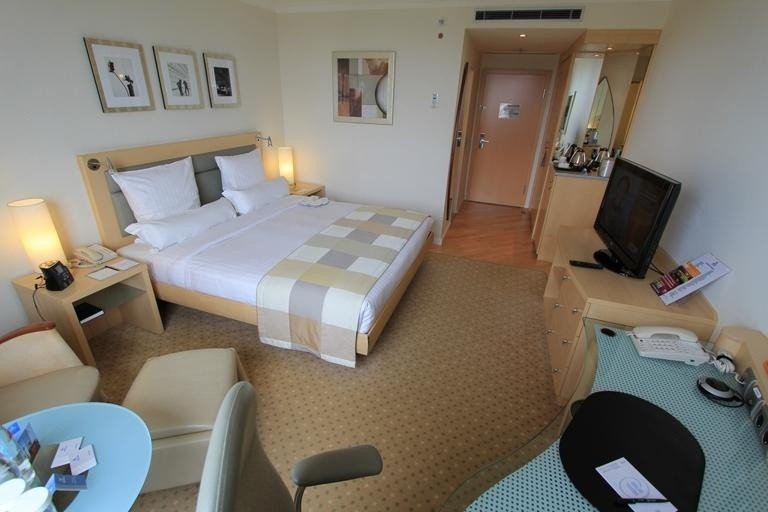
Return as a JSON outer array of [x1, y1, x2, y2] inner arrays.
[[630, 324, 712, 365], [72, 243, 118, 265]]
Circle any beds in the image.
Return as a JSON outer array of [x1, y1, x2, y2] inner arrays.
[[74, 131, 434, 368]]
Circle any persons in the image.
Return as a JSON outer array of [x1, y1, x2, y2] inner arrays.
[[177, 79, 190, 95]]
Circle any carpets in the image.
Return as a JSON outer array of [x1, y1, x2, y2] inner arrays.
[[91, 251, 559, 512]]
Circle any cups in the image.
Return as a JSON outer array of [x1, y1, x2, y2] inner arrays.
[[0, 477, 58, 512]]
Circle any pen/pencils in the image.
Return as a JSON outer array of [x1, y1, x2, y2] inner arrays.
[[620, 498, 670, 504]]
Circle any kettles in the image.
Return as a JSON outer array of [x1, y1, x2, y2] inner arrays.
[[565, 144, 578, 161], [568, 148, 586, 167], [595, 147, 610, 163]]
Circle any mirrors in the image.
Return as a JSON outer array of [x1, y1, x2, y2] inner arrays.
[[587, 76, 615, 149]]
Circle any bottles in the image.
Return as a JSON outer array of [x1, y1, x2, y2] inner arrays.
[[0, 426, 36, 486]]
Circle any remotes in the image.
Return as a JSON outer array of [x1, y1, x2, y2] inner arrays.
[[569, 259, 605, 271]]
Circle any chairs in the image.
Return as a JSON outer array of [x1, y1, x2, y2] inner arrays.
[[0, 322, 101, 424], [195, 382, 383, 512]]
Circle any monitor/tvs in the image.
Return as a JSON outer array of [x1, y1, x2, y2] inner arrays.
[[592, 156, 682, 279]]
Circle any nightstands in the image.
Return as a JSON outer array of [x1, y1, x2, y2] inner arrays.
[[12, 256, 164, 365], [293, 183, 326, 196]]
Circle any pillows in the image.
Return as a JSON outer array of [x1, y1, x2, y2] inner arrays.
[[219, 175, 290, 217], [111, 156, 202, 223], [123, 196, 236, 250], [214, 147, 264, 191]]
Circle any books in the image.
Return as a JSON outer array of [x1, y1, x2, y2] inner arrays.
[[74, 302, 105, 325]]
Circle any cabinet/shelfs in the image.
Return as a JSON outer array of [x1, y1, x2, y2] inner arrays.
[[530, 162, 610, 263], [542, 225, 718, 406]]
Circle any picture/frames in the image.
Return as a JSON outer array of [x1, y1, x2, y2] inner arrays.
[[151, 44, 204, 110], [560, 91, 576, 134], [83, 38, 158, 112], [332, 51, 395, 125], [202, 51, 241, 109]]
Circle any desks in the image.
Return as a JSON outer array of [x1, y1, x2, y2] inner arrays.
[[0, 402, 152, 512], [461, 318, 768, 512]]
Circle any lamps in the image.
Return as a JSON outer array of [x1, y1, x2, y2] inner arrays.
[[86, 155, 116, 177], [9, 198, 67, 273], [256, 135, 273, 149], [278, 146, 295, 187]]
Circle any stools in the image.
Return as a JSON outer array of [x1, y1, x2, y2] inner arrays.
[[124, 346, 249, 493]]
